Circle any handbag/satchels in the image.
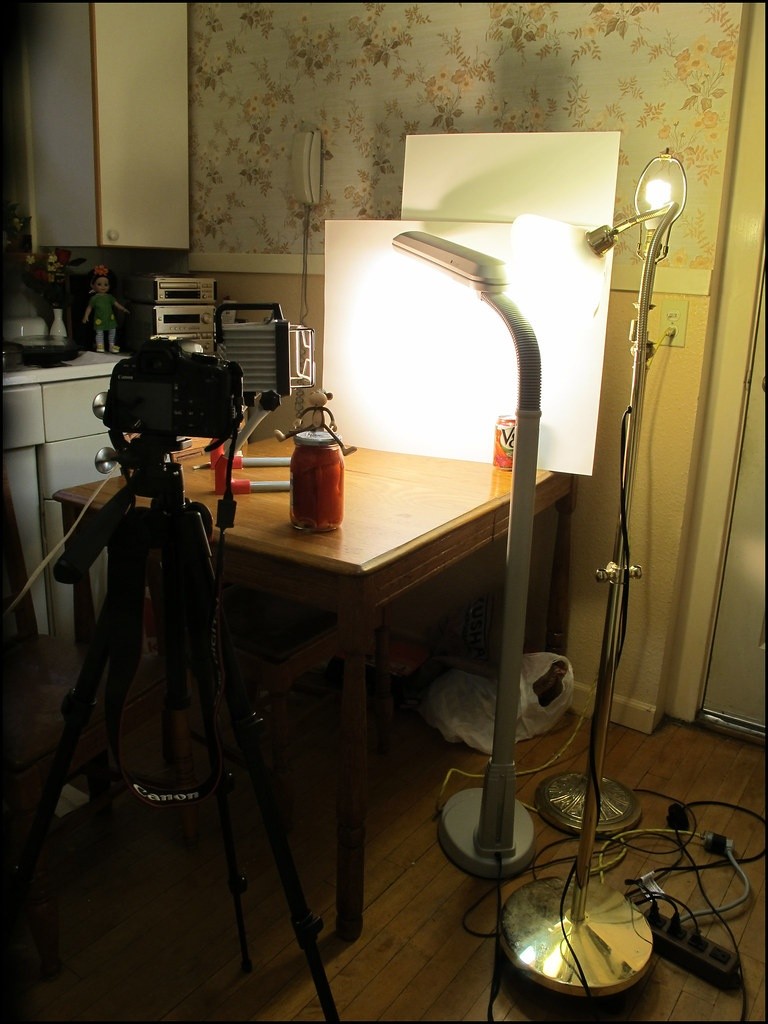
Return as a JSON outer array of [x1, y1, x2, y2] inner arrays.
[[417, 648, 575, 755]]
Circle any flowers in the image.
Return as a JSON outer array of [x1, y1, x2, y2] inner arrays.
[[2, 200, 31, 251], [93, 265, 110, 276], [24, 247, 89, 309]]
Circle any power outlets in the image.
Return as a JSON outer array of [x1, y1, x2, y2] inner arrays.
[[659, 299, 689, 348]]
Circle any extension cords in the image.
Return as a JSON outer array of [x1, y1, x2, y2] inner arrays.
[[642, 908, 740, 990]]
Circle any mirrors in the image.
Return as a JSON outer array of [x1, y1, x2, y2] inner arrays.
[[0, 3, 33, 254]]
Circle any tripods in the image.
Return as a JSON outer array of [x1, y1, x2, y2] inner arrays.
[[0, 461, 341, 1024]]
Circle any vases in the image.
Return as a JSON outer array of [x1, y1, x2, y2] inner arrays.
[[51, 309, 67, 337]]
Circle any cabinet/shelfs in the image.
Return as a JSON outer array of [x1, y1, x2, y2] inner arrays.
[[0, 375, 123, 647], [20, 2, 189, 247]]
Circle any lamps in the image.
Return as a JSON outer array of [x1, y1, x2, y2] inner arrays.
[[208, 303, 316, 493], [536, 155, 683, 834], [391, 230, 536, 876], [497, 202, 679, 999]]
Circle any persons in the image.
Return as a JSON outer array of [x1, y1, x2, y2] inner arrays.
[[81, 264, 131, 354]]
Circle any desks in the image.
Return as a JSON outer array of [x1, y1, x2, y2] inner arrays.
[[52, 430, 576, 937]]
